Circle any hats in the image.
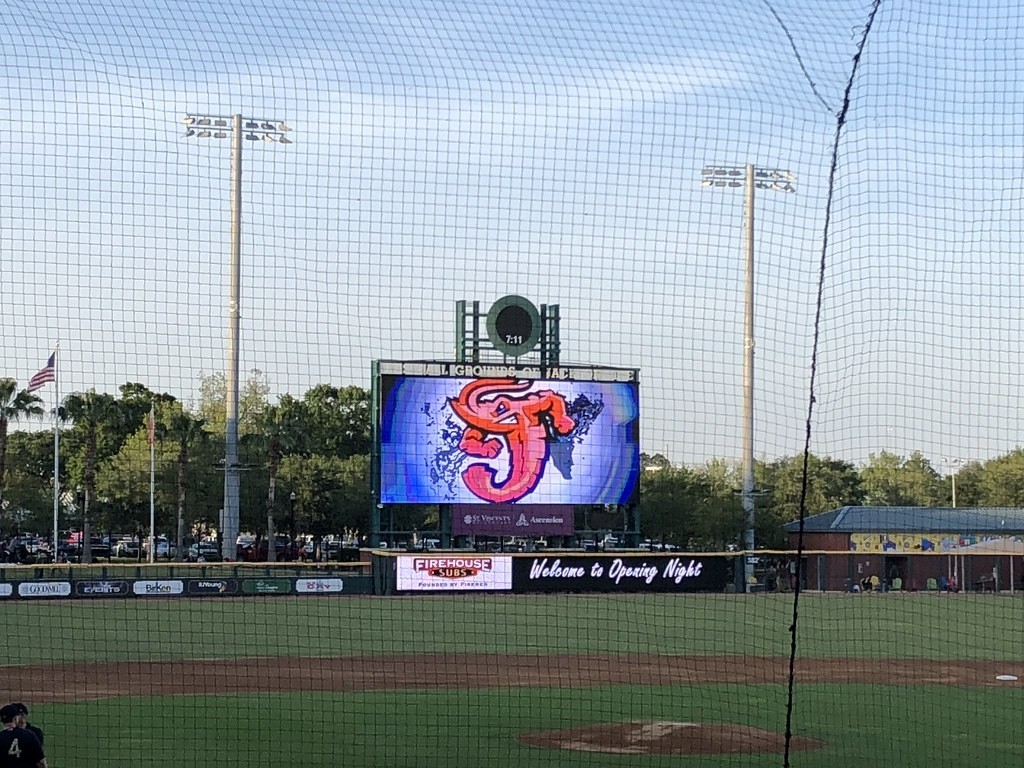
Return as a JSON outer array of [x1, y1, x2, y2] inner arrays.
[[0, 705, 17, 722], [12, 703, 28, 715]]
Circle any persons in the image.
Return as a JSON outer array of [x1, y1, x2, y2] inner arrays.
[[766, 557, 800, 595], [948, 575, 956, 586], [8, 535, 22, 563], [0, 705, 48, 768], [298, 542, 308, 563], [197, 553, 210, 569], [842, 573, 874, 593], [0, 702, 45, 745], [889, 564, 899, 578]]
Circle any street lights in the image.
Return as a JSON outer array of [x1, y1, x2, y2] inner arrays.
[[181, 112, 294, 561], [940, 458, 965, 508], [699, 165, 799, 582]]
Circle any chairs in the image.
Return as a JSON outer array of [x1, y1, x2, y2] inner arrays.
[[927, 577, 941, 594], [888, 578, 903, 593]]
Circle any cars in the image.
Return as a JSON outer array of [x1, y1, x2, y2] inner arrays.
[[0, 532, 682, 562]]
